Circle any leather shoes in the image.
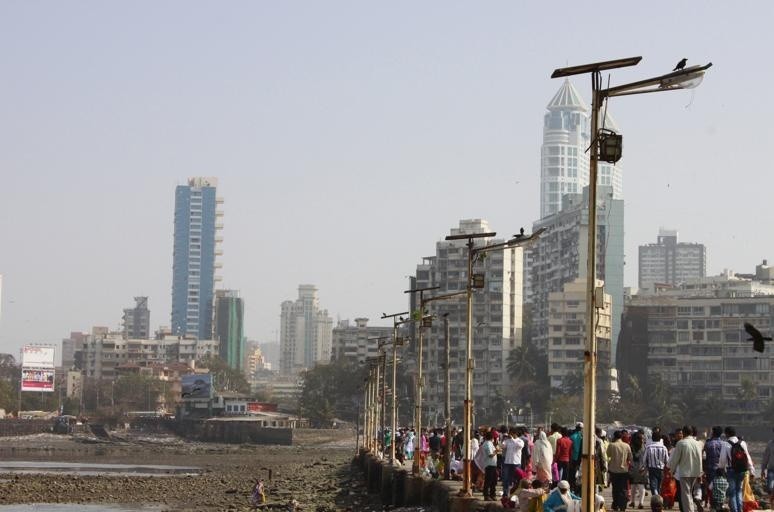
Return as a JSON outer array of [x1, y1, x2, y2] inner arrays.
[[484, 495, 497, 501]]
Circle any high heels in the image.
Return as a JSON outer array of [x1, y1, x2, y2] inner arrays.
[[629, 502, 635, 509], [638, 503, 643, 509]]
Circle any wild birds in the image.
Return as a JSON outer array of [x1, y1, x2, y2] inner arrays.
[[743, 321, 773, 354], [673, 57, 688, 72]]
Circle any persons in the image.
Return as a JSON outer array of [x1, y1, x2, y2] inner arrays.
[[379, 420, 773, 512], [252, 478, 265, 504]]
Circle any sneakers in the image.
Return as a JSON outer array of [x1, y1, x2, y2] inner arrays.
[[694, 498, 704, 512]]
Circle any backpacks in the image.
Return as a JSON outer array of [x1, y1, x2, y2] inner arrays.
[[725, 439, 748, 473]]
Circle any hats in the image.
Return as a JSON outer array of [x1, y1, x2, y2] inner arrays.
[[558, 480, 571, 490]]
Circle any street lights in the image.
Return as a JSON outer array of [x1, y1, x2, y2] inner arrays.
[[400, 278, 489, 480], [440, 223, 550, 504], [549, 54, 716, 512], [362, 306, 452, 470]]
